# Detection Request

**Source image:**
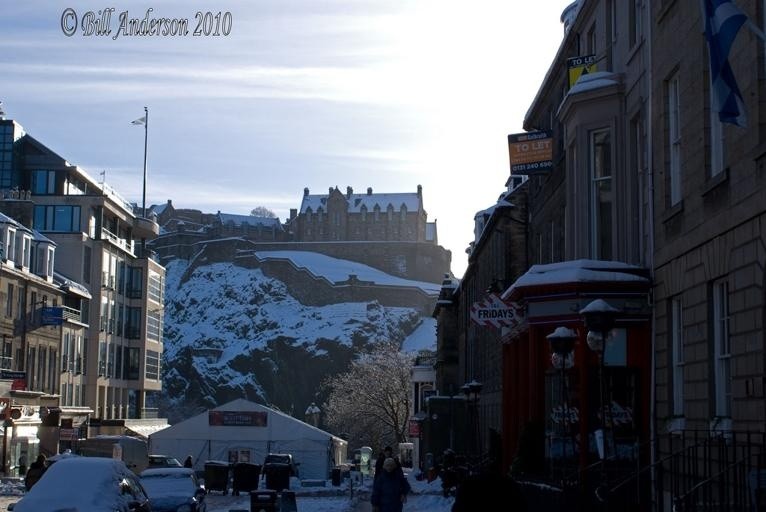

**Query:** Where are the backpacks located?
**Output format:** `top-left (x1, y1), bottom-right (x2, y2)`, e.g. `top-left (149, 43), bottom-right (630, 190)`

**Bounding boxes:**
top-left (25, 464), bottom-right (46, 489)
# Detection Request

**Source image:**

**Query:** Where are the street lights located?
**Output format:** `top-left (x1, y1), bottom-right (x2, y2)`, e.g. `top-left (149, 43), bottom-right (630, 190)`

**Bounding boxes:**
top-left (547, 327), bottom-right (580, 457)
top-left (461, 380), bottom-right (483, 463)
top-left (580, 299), bottom-right (620, 460)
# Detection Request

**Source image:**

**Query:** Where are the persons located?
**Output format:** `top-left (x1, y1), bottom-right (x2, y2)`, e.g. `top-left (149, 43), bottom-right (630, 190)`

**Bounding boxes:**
top-left (24, 454), bottom-right (48, 492)
top-left (183, 455), bottom-right (192, 468)
top-left (374, 446), bottom-right (393, 488)
top-left (369, 456), bottom-right (411, 512)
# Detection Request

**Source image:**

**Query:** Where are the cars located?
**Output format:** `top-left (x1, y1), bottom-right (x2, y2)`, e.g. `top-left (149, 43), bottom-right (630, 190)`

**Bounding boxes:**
top-left (8, 454), bottom-right (206, 512)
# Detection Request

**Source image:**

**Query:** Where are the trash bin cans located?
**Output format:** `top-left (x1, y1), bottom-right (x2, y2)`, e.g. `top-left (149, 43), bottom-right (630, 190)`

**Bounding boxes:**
top-left (232, 462), bottom-right (261, 496)
top-left (332, 469), bottom-right (340, 486)
top-left (251, 491), bottom-right (298, 512)
top-left (265, 463), bottom-right (289, 491)
top-left (204, 461), bottom-right (230, 496)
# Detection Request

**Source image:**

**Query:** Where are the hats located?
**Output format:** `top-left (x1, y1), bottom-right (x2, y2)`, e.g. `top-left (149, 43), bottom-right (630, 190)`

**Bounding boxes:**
top-left (384, 458), bottom-right (395, 467)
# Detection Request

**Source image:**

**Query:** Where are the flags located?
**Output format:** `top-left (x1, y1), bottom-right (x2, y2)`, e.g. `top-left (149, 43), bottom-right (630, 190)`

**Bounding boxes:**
top-left (693, 1), bottom-right (753, 129)
